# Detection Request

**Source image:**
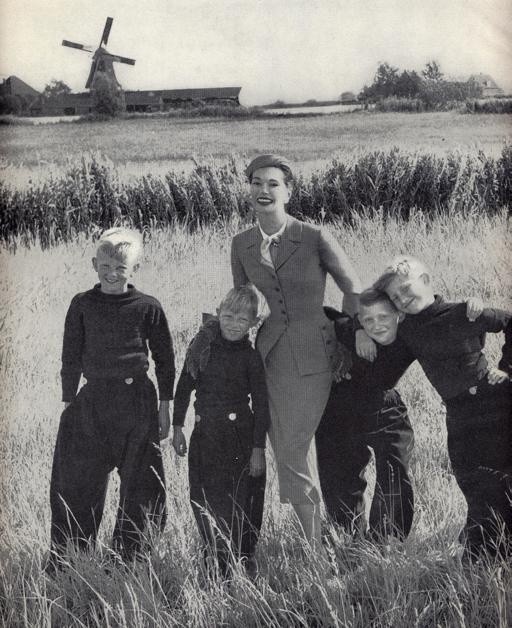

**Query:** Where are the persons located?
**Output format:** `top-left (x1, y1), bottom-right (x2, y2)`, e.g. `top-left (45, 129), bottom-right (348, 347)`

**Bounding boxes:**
top-left (314, 286), bottom-right (486, 560)
top-left (37, 223), bottom-right (176, 578)
top-left (167, 284), bottom-right (273, 579)
top-left (331, 256), bottom-right (512, 568)
top-left (182, 151), bottom-right (365, 578)
